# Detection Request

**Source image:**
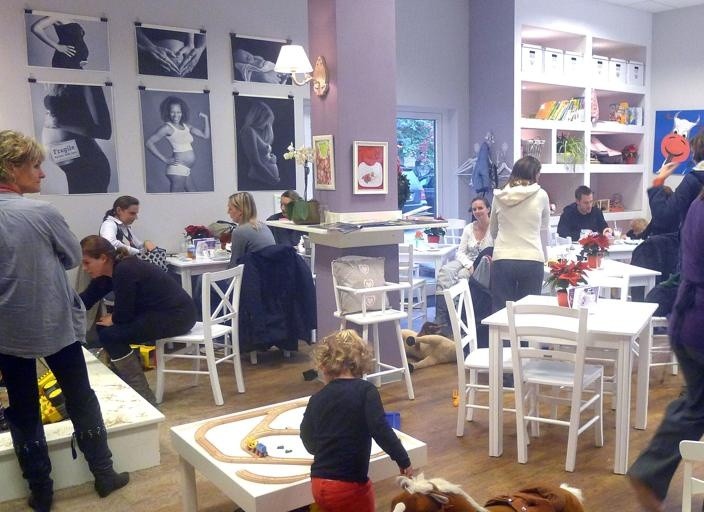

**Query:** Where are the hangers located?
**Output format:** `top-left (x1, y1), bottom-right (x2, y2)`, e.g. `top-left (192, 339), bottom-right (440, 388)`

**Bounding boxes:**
top-left (456, 153), bottom-right (513, 186)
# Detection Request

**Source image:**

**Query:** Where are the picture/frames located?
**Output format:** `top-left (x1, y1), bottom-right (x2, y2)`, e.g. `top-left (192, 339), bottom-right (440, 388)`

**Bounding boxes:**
top-left (353, 140), bottom-right (389, 195)
top-left (312, 135), bottom-right (335, 191)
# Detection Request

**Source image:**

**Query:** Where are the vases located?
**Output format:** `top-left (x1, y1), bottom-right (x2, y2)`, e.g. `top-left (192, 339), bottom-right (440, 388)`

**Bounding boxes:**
top-left (304, 161), bottom-right (315, 201)
top-left (624, 157), bottom-right (636, 164)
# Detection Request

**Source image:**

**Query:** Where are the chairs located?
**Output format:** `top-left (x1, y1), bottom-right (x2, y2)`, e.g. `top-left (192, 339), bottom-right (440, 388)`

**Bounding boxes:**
top-left (632, 315), bottom-right (679, 376)
top-left (680, 441), bottom-right (704, 511)
top-left (99, 297), bottom-right (115, 317)
top-left (444, 279), bottom-right (541, 446)
top-left (506, 300), bottom-right (605, 472)
top-left (442, 220), bottom-right (466, 245)
top-left (154, 264), bottom-right (246, 407)
top-left (330, 255), bottom-right (415, 399)
top-left (398, 245), bottom-right (427, 330)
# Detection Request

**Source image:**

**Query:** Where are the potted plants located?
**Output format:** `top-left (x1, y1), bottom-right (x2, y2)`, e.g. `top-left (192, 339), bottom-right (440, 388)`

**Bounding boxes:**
top-left (556, 131), bottom-right (584, 164)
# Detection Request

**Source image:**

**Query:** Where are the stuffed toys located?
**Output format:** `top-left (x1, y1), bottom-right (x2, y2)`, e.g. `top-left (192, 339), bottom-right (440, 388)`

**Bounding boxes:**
top-left (403, 327), bottom-right (464, 371)
top-left (393, 474), bottom-right (585, 511)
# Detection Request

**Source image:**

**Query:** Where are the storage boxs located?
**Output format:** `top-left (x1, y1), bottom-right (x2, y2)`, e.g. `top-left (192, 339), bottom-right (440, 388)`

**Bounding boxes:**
top-left (522, 138), bottom-right (545, 163)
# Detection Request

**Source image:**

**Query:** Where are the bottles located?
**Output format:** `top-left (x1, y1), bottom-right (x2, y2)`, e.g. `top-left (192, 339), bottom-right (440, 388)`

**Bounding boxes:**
top-left (545, 240), bottom-right (574, 262)
top-left (182, 241), bottom-right (223, 262)
top-left (584, 227), bottom-right (626, 245)
top-left (616, 106), bottom-right (623, 122)
top-left (582, 287), bottom-right (597, 315)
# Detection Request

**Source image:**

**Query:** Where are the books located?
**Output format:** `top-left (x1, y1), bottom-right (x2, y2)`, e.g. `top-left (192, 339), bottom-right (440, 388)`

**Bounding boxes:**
top-left (537, 97), bottom-right (585, 122)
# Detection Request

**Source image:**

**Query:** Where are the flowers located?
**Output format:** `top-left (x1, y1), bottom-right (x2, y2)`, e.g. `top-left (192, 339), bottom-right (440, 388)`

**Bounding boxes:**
top-left (184, 224), bottom-right (213, 238)
top-left (622, 143), bottom-right (639, 160)
top-left (283, 141), bottom-right (316, 201)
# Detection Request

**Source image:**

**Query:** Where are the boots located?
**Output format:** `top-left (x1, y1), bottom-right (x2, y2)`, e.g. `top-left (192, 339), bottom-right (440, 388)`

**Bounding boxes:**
top-left (26, 475), bottom-right (54, 512)
top-left (92, 466), bottom-right (131, 500)
top-left (110, 347), bottom-right (157, 405)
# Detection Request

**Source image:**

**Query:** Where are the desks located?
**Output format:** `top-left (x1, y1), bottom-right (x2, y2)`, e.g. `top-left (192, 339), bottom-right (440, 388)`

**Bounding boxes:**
top-left (544, 244), bottom-right (663, 301)
top-left (404, 242), bottom-right (459, 304)
top-left (165, 252), bottom-right (232, 355)
top-left (480, 293), bottom-right (659, 475)
top-left (265, 220), bottom-right (449, 373)
top-left (169, 394), bottom-right (428, 512)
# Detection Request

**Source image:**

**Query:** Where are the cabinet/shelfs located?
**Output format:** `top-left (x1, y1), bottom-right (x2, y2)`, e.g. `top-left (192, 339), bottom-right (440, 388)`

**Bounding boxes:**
top-left (470, 0), bottom-right (651, 241)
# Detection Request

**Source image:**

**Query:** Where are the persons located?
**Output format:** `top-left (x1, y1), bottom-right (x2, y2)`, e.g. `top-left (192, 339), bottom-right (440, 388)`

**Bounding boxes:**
top-left (624, 128), bottom-right (704, 512)
top-left (489, 156), bottom-right (551, 313)
top-left (135, 21), bottom-right (208, 76)
top-left (100, 196), bottom-right (155, 300)
top-left (75, 235), bottom-right (197, 407)
top-left (147, 93), bottom-right (210, 192)
top-left (430, 197), bottom-right (495, 329)
top-left (31, 12), bottom-right (88, 68)
top-left (235, 45), bottom-right (280, 84)
top-left (41, 84), bottom-right (113, 193)
top-left (558, 186), bottom-right (614, 243)
top-left (241, 99), bottom-right (281, 190)
top-left (194, 191), bottom-right (275, 306)
top-left (299, 329), bottom-right (413, 512)
top-left (0, 127), bottom-right (129, 512)
top-left (266, 190), bottom-right (304, 246)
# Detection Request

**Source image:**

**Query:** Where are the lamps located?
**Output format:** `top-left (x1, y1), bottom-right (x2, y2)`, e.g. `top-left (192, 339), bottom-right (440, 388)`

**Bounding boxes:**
top-left (275, 43), bottom-right (328, 98)
